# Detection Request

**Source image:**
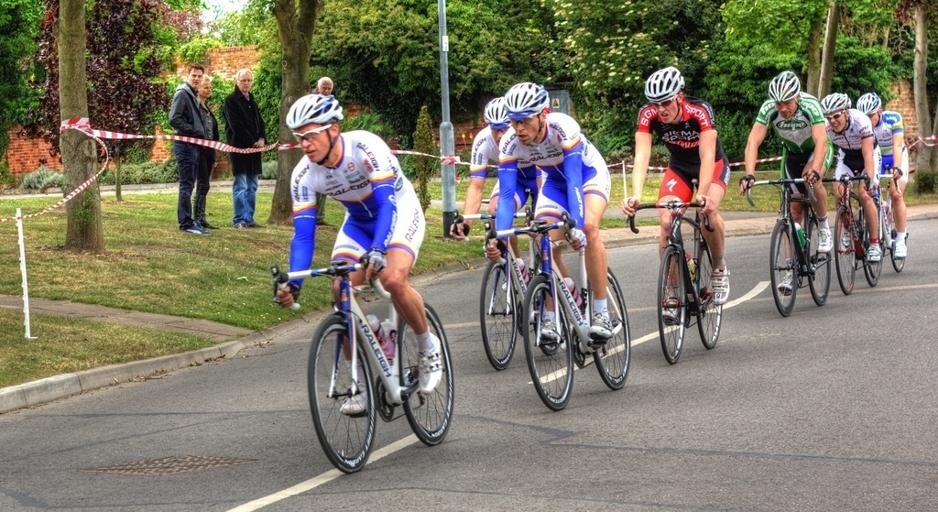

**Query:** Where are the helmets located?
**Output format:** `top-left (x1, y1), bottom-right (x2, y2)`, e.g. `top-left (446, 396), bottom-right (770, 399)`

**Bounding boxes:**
top-left (820, 93), bottom-right (852, 114)
top-left (768, 70), bottom-right (801, 103)
top-left (856, 91), bottom-right (882, 115)
top-left (483, 81), bottom-right (551, 129)
top-left (645, 66), bottom-right (685, 104)
top-left (285, 93), bottom-right (344, 130)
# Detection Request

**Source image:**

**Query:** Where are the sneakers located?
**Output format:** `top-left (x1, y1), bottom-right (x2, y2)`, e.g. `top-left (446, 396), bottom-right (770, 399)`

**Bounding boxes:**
top-left (895, 238), bottom-right (907, 257)
top-left (817, 229), bottom-right (833, 253)
top-left (234, 222), bottom-right (266, 230)
top-left (662, 296), bottom-right (680, 320)
top-left (541, 319), bottom-right (556, 339)
top-left (418, 333), bottom-right (442, 392)
top-left (777, 271), bottom-right (792, 296)
top-left (710, 266), bottom-right (730, 305)
top-left (842, 229), bottom-right (852, 250)
top-left (867, 245), bottom-right (881, 261)
top-left (186, 221), bottom-right (209, 234)
top-left (340, 383), bottom-right (367, 415)
top-left (589, 315), bottom-right (613, 339)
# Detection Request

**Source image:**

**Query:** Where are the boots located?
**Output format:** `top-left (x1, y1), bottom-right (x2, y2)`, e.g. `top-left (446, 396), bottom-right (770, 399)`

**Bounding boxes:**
top-left (193, 194), bottom-right (219, 233)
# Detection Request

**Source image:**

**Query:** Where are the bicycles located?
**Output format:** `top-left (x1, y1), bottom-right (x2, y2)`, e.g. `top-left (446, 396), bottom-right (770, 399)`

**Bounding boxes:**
top-left (449, 205), bottom-right (566, 371)
top-left (484, 211), bottom-right (633, 410)
top-left (742, 174), bottom-right (832, 318)
top-left (629, 198), bottom-right (724, 363)
top-left (820, 170), bottom-right (910, 296)
top-left (270, 254), bottom-right (453, 473)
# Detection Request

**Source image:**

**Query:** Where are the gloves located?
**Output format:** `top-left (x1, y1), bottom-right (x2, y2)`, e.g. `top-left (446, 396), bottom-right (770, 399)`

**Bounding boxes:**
top-left (803, 170), bottom-right (820, 185)
top-left (487, 237), bottom-right (508, 265)
top-left (565, 226), bottom-right (587, 251)
top-left (738, 174), bottom-right (755, 191)
top-left (890, 166), bottom-right (903, 179)
top-left (361, 248), bottom-right (385, 275)
top-left (449, 221), bottom-right (470, 242)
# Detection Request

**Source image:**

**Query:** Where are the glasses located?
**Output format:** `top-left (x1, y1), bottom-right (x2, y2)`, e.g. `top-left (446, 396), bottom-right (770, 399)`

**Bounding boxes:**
top-left (652, 95), bottom-right (678, 107)
top-left (292, 124), bottom-right (333, 140)
top-left (825, 110), bottom-right (847, 120)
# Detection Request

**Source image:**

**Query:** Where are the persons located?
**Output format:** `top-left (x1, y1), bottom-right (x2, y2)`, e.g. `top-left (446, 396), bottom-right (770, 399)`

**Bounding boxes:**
top-left (483, 81), bottom-right (614, 341)
top-left (737, 69), bottom-right (835, 292)
top-left (274, 92), bottom-right (446, 417)
top-left (620, 65), bottom-right (732, 320)
top-left (448, 96), bottom-right (581, 311)
top-left (166, 62), bottom-right (214, 238)
top-left (820, 92), bottom-right (884, 265)
top-left (286, 77), bottom-right (334, 226)
top-left (193, 73), bottom-right (222, 230)
top-left (220, 67), bottom-right (269, 230)
top-left (855, 91), bottom-right (911, 259)
